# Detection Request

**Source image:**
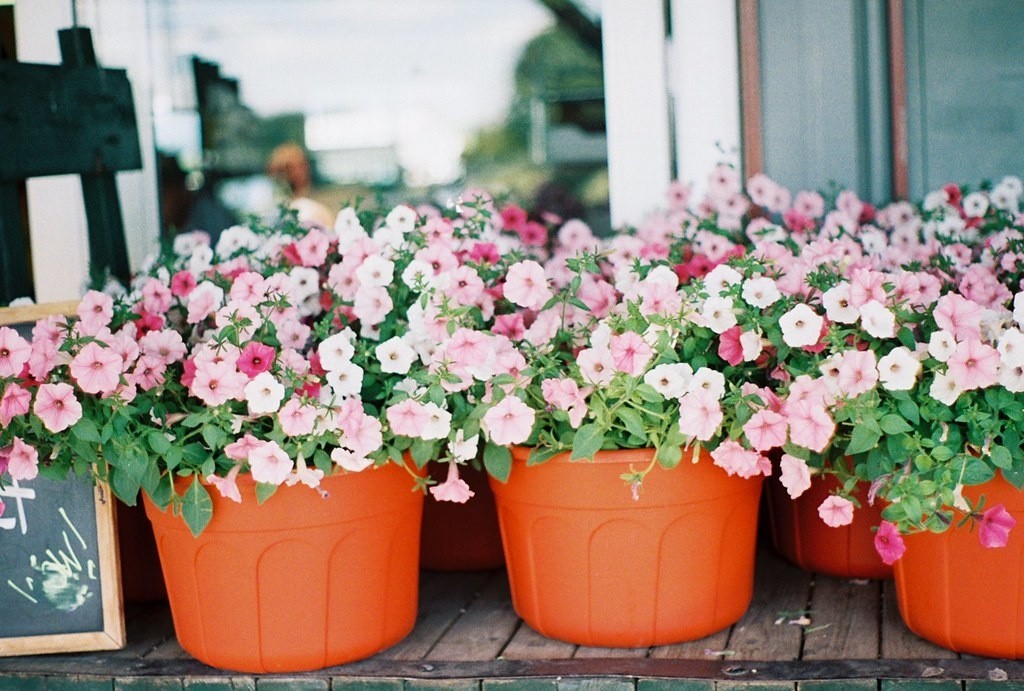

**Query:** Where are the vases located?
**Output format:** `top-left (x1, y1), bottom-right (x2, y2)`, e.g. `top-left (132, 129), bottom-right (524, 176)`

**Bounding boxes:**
top-left (114, 444), bottom-right (1024, 674)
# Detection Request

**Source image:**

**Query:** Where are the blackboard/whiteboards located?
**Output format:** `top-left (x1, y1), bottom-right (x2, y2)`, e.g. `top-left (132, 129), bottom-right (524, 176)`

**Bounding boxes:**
top-left (0, 300), bottom-right (125, 659)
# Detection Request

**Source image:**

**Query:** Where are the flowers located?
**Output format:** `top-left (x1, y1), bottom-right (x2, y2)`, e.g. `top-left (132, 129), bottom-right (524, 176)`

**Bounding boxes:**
top-left (0, 173), bottom-right (1024, 565)
